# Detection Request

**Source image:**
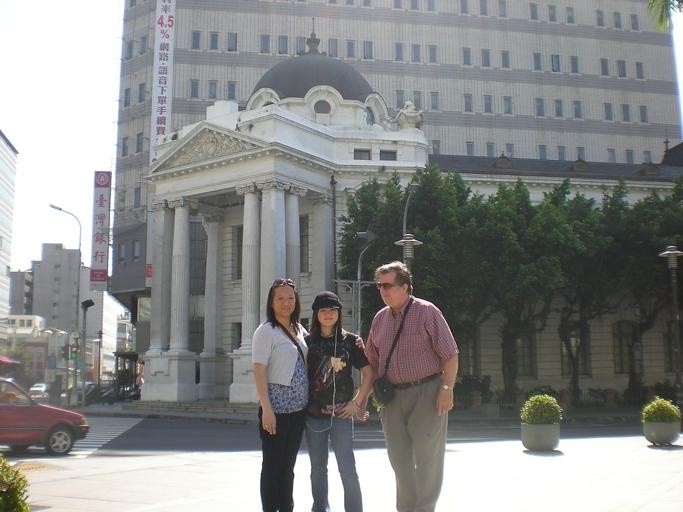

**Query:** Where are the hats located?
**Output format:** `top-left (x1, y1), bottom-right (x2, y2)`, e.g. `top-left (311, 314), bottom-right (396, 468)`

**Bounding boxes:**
top-left (311, 291), bottom-right (342, 309)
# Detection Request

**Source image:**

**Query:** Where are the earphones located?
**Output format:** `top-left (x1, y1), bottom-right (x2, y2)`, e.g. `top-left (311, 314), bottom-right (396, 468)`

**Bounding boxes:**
top-left (332, 410), bottom-right (335, 417)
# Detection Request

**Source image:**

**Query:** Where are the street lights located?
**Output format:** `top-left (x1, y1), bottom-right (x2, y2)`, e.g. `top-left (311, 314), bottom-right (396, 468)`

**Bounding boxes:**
top-left (400, 183), bottom-right (421, 237)
top-left (393, 232), bottom-right (422, 297)
top-left (49, 203), bottom-right (83, 406)
top-left (80, 298), bottom-right (94, 404)
top-left (658, 242), bottom-right (682, 412)
top-left (93, 327), bottom-right (104, 393)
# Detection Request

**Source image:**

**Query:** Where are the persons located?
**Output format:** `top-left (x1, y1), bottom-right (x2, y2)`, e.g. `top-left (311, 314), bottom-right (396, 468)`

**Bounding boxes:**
top-left (252, 278), bottom-right (365, 512)
top-left (304, 291), bottom-right (373, 512)
top-left (356, 260), bottom-right (460, 512)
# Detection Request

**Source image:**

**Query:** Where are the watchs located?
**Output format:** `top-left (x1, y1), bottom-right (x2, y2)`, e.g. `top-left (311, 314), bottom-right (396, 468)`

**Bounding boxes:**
top-left (440, 384), bottom-right (453, 391)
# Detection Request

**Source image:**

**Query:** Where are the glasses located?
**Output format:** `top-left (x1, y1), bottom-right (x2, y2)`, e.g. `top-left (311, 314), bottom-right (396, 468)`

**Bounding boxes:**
top-left (272, 278), bottom-right (296, 289)
top-left (375, 282), bottom-right (401, 289)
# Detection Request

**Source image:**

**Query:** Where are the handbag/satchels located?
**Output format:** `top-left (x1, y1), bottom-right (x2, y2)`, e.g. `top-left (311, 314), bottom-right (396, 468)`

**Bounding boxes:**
top-left (371, 379), bottom-right (394, 406)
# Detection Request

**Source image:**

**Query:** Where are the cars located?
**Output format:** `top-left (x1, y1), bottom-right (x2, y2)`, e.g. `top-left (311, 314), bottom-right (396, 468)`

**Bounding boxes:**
top-left (28, 377), bottom-right (118, 401)
top-left (0, 377), bottom-right (89, 456)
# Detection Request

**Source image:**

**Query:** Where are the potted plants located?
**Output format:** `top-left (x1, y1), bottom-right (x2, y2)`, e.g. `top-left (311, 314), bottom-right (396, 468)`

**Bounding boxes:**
top-left (520, 394), bottom-right (563, 451)
top-left (641, 397), bottom-right (682, 445)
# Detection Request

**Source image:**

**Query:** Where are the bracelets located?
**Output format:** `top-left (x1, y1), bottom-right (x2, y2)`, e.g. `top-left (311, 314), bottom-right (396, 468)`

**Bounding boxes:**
top-left (352, 399), bottom-right (360, 409)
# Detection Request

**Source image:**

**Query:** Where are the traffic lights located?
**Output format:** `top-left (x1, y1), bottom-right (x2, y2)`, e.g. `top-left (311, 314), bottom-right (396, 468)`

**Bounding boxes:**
top-left (70, 352), bottom-right (77, 359)
top-left (60, 344), bottom-right (67, 359)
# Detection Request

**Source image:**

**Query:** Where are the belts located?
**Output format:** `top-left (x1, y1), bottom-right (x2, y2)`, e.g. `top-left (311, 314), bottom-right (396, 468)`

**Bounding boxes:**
top-left (393, 370), bottom-right (443, 391)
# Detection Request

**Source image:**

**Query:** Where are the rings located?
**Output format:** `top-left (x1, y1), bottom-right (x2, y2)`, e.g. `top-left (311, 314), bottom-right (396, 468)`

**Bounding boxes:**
top-left (269, 424), bottom-right (272, 426)
